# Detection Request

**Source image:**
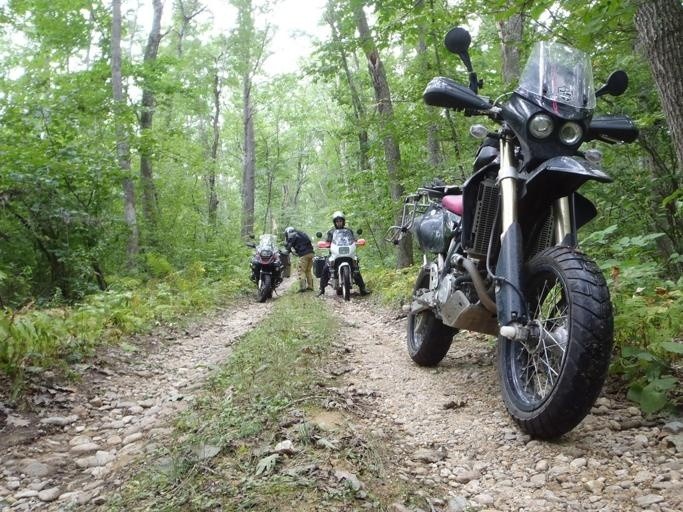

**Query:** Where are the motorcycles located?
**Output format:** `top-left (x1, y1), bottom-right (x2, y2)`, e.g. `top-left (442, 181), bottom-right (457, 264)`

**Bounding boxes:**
top-left (382, 21), bottom-right (650, 446)
top-left (310, 228), bottom-right (367, 303)
top-left (244, 233), bottom-right (290, 305)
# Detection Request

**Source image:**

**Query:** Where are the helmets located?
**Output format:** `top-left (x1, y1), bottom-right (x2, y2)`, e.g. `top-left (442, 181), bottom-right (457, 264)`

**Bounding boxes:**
top-left (333, 211), bottom-right (345, 229)
top-left (284, 227), bottom-right (295, 236)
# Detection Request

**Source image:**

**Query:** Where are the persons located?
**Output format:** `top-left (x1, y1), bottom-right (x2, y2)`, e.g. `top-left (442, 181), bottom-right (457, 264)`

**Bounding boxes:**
top-left (310, 210), bottom-right (372, 297)
top-left (282, 226), bottom-right (315, 294)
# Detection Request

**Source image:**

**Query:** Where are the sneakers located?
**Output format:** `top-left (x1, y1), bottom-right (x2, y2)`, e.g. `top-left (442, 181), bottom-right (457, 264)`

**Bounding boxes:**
top-left (360, 288), bottom-right (370, 295)
top-left (316, 290), bottom-right (324, 296)
